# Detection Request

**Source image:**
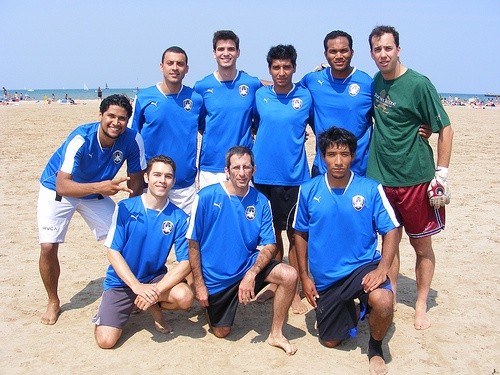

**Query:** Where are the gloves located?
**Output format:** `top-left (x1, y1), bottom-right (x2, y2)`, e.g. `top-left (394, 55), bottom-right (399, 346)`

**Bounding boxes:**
top-left (426, 165), bottom-right (451, 207)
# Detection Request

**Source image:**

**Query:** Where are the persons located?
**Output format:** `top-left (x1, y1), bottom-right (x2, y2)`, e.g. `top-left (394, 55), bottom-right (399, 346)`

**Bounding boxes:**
top-left (0, 84), bottom-right (76, 109)
top-left (37, 26), bottom-right (454, 374)
top-left (97, 86), bottom-right (102, 101)
top-left (433, 93), bottom-right (500, 112)
top-left (119, 91), bottom-right (134, 101)
